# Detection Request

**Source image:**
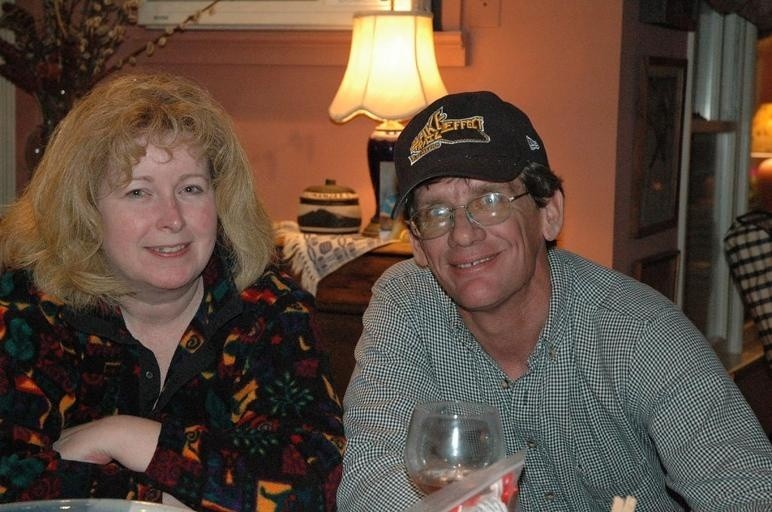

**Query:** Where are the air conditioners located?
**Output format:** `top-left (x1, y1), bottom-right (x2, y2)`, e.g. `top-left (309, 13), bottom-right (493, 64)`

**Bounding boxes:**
top-left (137, 0), bottom-right (429, 32)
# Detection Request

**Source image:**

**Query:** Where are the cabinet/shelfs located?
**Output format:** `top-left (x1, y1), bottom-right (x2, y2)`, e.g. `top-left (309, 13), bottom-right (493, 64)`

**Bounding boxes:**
top-left (271, 218), bottom-right (420, 402)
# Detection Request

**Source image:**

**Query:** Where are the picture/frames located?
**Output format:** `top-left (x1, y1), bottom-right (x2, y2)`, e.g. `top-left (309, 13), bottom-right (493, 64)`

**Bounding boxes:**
top-left (633, 249), bottom-right (680, 307)
top-left (630, 54), bottom-right (688, 241)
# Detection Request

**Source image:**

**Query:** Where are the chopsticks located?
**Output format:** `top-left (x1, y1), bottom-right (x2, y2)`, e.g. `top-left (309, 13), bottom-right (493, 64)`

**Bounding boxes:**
top-left (610, 495), bottom-right (638, 511)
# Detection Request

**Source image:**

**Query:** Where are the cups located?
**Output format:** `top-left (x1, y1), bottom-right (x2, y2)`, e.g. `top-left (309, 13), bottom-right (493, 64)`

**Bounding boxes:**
top-left (402, 400), bottom-right (505, 512)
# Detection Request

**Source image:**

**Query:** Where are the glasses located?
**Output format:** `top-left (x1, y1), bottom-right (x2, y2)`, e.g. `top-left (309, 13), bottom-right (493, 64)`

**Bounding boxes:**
top-left (409, 192), bottom-right (528, 240)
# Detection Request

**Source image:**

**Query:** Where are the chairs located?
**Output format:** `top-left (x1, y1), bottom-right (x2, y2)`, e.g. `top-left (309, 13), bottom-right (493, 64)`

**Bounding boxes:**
top-left (722, 211), bottom-right (772, 372)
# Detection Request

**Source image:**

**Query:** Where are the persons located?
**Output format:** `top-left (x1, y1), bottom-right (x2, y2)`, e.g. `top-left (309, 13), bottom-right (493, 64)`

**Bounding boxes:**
top-left (1, 69), bottom-right (347, 512)
top-left (337, 90), bottom-right (771, 512)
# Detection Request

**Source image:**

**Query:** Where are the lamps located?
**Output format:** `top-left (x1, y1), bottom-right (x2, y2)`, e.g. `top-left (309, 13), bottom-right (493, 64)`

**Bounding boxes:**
top-left (327, 10), bottom-right (449, 237)
top-left (750, 103), bottom-right (772, 210)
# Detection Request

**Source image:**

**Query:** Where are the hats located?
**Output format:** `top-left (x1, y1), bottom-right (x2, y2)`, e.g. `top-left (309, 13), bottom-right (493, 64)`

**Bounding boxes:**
top-left (391, 91), bottom-right (548, 220)
